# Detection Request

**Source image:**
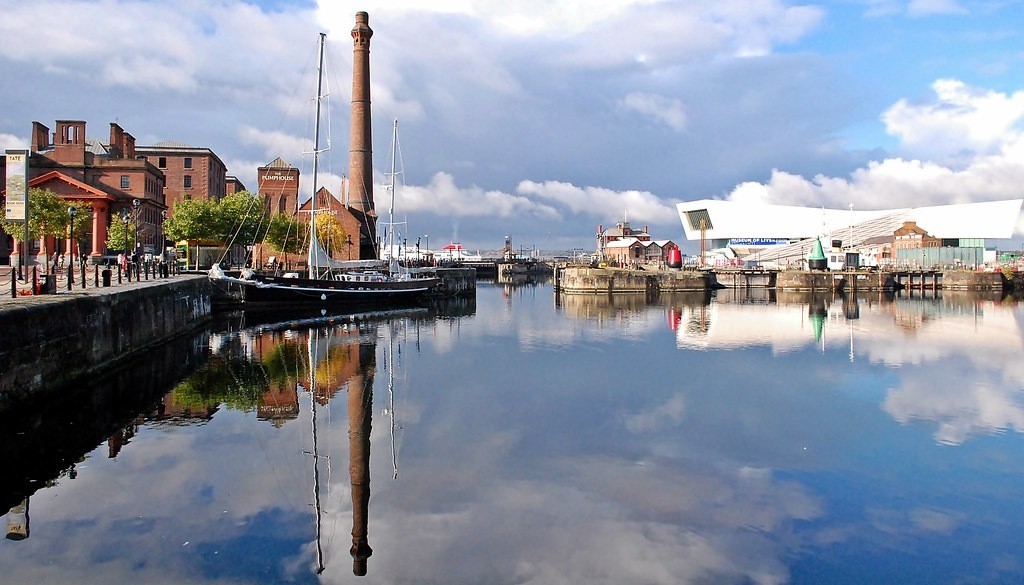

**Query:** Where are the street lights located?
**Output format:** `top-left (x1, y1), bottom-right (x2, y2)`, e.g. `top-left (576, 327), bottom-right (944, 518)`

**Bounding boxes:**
top-left (132, 199), bottom-right (141, 283)
top-left (66, 206), bottom-right (77, 290)
top-left (122, 206), bottom-right (131, 282)
top-left (161, 211), bottom-right (167, 276)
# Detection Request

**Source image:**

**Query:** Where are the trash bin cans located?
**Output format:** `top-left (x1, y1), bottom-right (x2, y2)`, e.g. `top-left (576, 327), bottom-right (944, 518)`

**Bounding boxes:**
top-left (41, 275), bottom-right (57, 294)
top-left (102, 269), bottom-right (113, 287)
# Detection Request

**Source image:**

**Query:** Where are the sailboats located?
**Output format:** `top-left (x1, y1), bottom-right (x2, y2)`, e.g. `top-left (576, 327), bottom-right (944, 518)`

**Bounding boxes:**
top-left (209, 30), bottom-right (441, 293)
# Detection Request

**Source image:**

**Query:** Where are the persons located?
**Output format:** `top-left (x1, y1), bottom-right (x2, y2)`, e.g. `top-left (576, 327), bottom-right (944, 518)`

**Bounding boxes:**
top-left (82, 252), bottom-right (88, 268)
top-left (396, 256), bottom-right (461, 267)
top-left (50, 251), bottom-right (59, 273)
top-left (117, 251), bottom-right (180, 274)
top-left (57, 252), bottom-right (64, 272)
